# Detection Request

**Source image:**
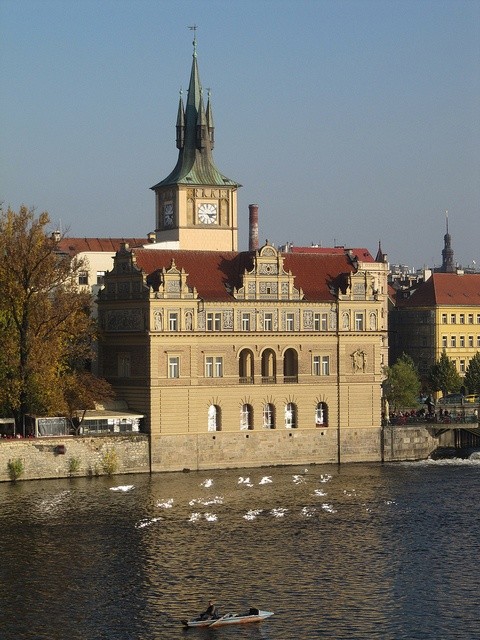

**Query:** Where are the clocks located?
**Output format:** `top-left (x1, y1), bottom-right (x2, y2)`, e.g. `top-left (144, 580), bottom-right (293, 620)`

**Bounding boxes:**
top-left (195, 200), bottom-right (218, 226)
top-left (163, 199), bottom-right (174, 225)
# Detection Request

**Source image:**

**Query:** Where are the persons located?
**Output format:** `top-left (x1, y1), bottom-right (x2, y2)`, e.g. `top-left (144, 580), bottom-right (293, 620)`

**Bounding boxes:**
top-left (199, 600), bottom-right (216, 620)
top-left (473, 409), bottom-right (478, 422)
top-left (388, 408), bottom-right (465, 424)
top-left (0, 431), bottom-right (34, 440)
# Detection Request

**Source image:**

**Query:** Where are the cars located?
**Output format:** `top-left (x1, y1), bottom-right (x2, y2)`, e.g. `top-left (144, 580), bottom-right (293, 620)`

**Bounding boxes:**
top-left (467, 394), bottom-right (479, 403)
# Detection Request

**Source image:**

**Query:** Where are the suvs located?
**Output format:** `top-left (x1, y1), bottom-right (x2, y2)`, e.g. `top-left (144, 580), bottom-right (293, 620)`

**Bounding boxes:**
top-left (439, 394), bottom-right (464, 403)
